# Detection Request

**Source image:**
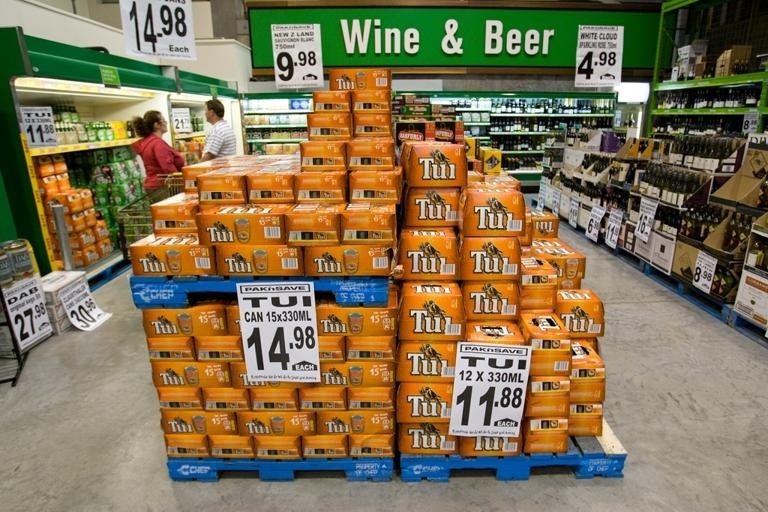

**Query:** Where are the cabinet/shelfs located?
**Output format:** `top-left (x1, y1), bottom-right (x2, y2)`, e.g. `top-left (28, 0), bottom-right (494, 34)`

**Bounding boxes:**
top-left (1, 27), bottom-right (247, 291)
top-left (239, 92), bottom-right (620, 187)
top-left (646, 0), bottom-right (768, 137)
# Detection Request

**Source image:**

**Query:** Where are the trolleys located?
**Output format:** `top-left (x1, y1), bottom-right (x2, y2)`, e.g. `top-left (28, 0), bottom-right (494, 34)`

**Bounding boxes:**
top-left (117, 173), bottom-right (184, 264)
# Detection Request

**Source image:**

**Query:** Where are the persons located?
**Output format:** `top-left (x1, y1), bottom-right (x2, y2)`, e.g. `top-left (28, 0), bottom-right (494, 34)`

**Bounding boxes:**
top-left (131, 111), bottom-right (184, 204)
top-left (200, 99), bottom-right (235, 162)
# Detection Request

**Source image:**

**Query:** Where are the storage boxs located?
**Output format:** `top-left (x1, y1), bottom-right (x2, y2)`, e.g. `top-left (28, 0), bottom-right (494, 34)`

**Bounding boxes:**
top-left (128, 69), bottom-right (607, 458)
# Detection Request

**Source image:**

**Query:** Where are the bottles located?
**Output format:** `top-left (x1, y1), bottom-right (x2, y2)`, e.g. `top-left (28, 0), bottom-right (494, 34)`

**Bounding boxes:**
top-left (489, 97), bottom-right (544, 169)
top-left (544, 59), bottom-right (767, 296)
top-left (126, 121), bottom-right (133, 137)
top-left (52, 105), bottom-right (113, 144)
